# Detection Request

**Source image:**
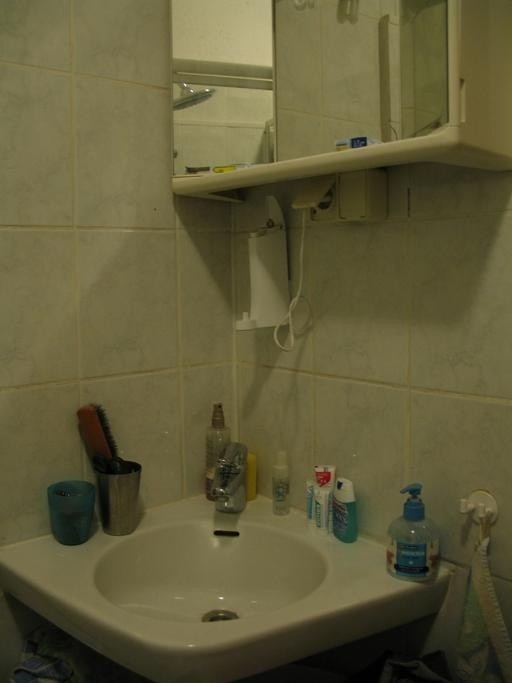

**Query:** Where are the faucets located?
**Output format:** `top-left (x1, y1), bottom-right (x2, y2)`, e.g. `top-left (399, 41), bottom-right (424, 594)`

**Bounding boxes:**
top-left (209, 440), bottom-right (247, 513)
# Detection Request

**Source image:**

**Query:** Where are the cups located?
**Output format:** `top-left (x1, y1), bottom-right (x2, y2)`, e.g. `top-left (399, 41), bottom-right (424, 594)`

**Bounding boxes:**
top-left (47, 480), bottom-right (95, 546)
top-left (96, 460), bottom-right (142, 536)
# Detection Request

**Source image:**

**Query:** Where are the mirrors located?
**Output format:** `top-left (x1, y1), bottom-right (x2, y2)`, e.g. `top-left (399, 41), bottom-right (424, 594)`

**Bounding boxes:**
top-left (169, 1), bottom-right (449, 177)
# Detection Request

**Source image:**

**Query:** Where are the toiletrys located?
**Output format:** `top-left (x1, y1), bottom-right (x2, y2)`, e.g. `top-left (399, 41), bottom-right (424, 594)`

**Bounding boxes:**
top-left (272, 450), bottom-right (290, 516)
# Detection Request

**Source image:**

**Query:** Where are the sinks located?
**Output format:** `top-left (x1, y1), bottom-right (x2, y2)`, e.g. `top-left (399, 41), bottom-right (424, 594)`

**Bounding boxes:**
top-left (94, 518), bottom-right (330, 622)
